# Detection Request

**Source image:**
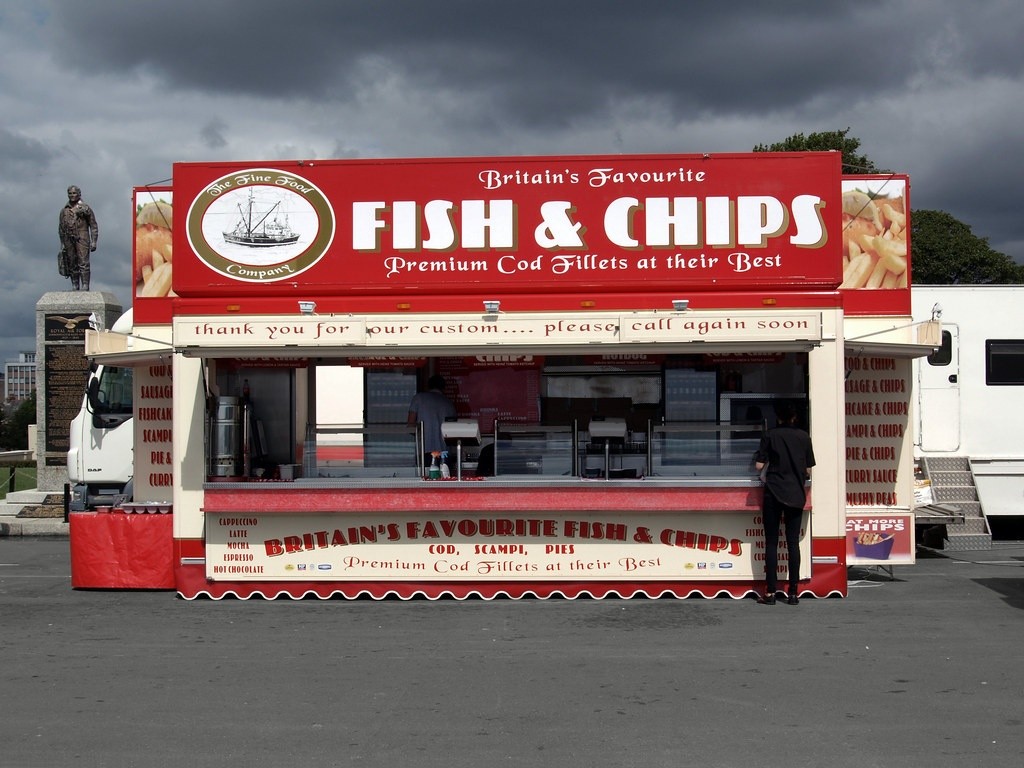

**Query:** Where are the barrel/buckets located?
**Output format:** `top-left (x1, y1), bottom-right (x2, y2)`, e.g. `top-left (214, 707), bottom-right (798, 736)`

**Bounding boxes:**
top-left (209, 395), bottom-right (243, 478)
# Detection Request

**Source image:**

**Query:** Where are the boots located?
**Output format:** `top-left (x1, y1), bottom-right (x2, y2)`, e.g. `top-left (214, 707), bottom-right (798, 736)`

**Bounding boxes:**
top-left (81, 271), bottom-right (91, 291)
top-left (72, 273), bottom-right (79, 290)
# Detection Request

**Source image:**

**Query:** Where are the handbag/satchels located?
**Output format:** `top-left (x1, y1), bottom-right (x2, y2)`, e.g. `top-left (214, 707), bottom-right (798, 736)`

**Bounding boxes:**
top-left (57, 248), bottom-right (69, 278)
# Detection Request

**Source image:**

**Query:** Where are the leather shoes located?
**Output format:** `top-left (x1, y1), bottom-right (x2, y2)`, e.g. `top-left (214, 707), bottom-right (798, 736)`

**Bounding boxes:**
top-left (758, 596), bottom-right (775, 604)
top-left (788, 595), bottom-right (800, 604)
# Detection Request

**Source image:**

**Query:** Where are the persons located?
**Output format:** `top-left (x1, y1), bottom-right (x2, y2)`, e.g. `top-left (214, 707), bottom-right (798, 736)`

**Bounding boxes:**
top-left (751, 398), bottom-right (817, 605)
top-left (57, 185), bottom-right (99, 292)
top-left (736, 403), bottom-right (768, 440)
top-left (406, 375), bottom-right (458, 478)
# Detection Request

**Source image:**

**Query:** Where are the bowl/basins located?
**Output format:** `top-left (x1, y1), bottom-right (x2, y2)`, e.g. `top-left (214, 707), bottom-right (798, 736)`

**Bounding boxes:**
top-left (852, 533), bottom-right (895, 560)
